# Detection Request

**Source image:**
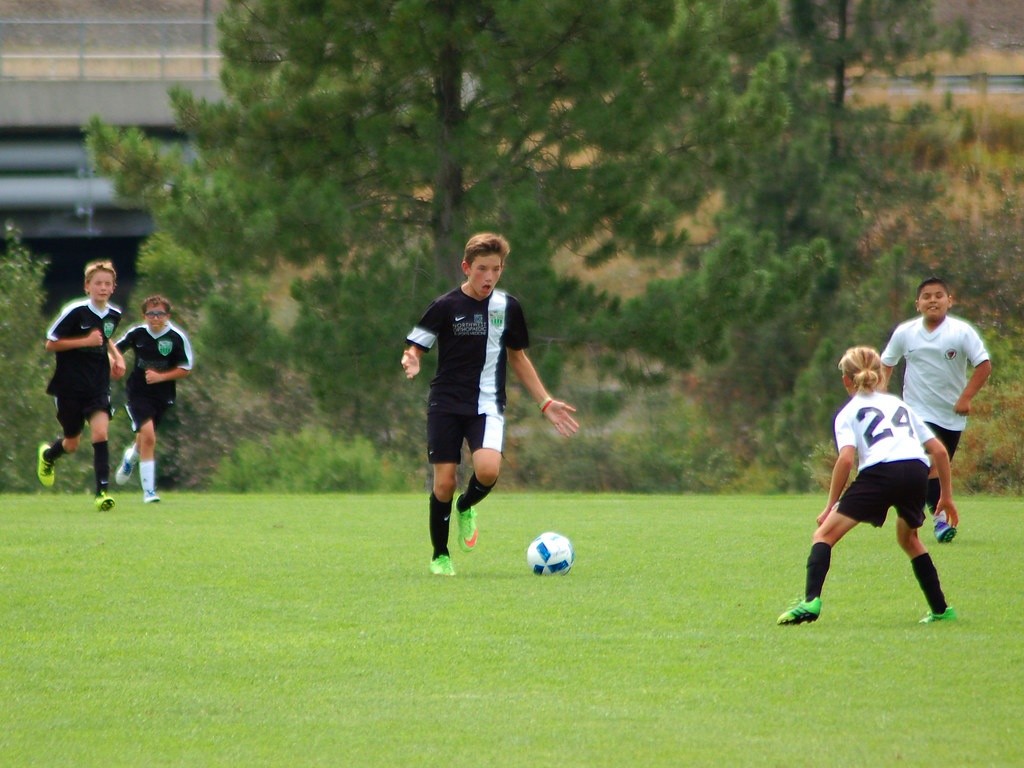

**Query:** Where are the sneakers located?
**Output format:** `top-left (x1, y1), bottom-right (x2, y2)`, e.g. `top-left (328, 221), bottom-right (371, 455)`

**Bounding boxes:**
top-left (144, 489), bottom-right (161, 503)
top-left (934, 521), bottom-right (957, 544)
top-left (429, 554), bottom-right (456, 576)
top-left (454, 494), bottom-right (477, 553)
top-left (37, 445), bottom-right (54, 487)
top-left (777, 597), bottom-right (822, 625)
top-left (918, 607), bottom-right (956, 623)
top-left (116, 448), bottom-right (137, 485)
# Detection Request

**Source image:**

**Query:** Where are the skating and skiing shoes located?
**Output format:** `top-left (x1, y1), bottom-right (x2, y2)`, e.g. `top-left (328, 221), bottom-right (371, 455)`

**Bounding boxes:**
top-left (96, 492), bottom-right (114, 510)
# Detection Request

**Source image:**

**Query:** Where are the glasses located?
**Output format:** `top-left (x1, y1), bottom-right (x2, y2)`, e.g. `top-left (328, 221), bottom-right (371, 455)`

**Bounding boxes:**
top-left (145, 311), bottom-right (167, 318)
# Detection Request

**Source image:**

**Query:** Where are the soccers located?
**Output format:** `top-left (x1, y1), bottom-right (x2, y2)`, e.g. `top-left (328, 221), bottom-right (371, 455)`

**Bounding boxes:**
top-left (525, 531), bottom-right (575, 577)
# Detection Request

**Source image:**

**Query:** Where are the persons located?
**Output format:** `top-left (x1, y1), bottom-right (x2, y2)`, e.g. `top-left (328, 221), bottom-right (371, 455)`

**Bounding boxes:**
top-left (108, 296), bottom-right (194, 503)
top-left (881, 278), bottom-right (991, 542)
top-left (401, 231), bottom-right (579, 575)
top-left (776, 346), bottom-right (958, 625)
top-left (38, 259), bottom-right (127, 511)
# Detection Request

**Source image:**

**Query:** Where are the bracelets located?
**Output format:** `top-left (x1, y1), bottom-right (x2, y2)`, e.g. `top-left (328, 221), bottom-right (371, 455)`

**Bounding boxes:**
top-left (537, 397), bottom-right (552, 413)
